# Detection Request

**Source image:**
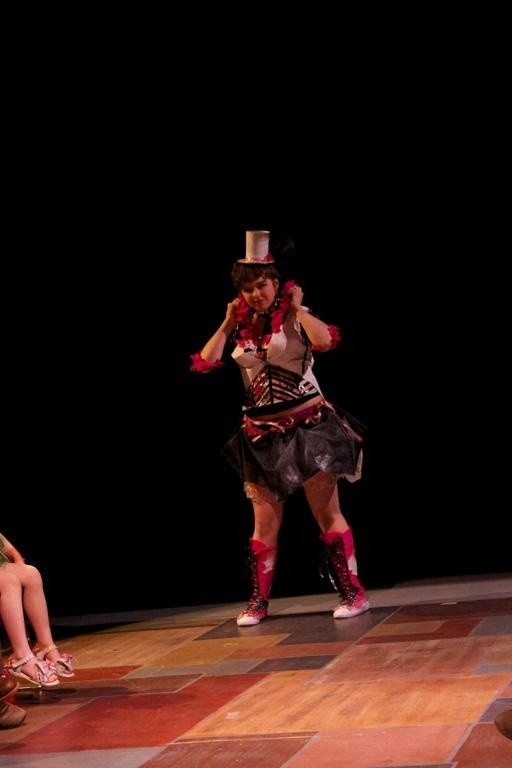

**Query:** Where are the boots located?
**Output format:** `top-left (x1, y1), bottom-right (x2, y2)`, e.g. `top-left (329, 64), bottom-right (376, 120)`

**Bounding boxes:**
top-left (318, 526), bottom-right (371, 620)
top-left (235, 538), bottom-right (278, 626)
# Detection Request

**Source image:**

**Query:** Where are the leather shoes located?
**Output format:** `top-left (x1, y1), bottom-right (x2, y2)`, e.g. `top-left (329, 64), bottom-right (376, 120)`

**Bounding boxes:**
top-left (0, 674), bottom-right (20, 703)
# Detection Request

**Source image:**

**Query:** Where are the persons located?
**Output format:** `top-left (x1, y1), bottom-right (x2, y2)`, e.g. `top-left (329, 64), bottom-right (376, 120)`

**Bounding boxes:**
top-left (1, 531), bottom-right (79, 690)
top-left (184, 261), bottom-right (370, 627)
top-left (0, 671), bottom-right (29, 730)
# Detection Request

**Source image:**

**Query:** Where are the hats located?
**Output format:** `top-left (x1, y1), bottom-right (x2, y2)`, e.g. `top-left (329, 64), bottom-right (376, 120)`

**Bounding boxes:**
top-left (237, 229), bottom-right (278, 264)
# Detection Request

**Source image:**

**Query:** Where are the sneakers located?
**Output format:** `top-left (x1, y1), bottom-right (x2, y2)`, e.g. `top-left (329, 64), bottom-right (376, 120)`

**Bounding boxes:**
top-left (0, 699), bottom-right (27, 730)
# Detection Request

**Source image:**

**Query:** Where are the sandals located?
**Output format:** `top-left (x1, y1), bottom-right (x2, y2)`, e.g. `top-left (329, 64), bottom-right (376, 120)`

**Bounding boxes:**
top-left (31, 644), bottom-right (75, 678)
top-left (5, 653), bottom-right (60, 687)
